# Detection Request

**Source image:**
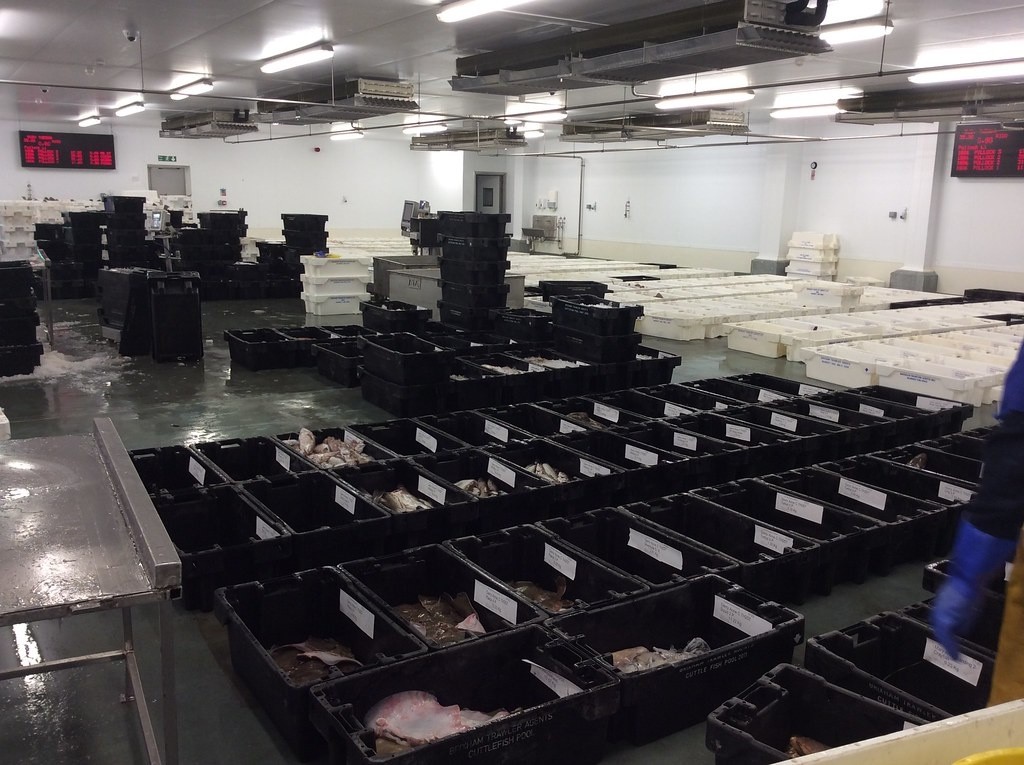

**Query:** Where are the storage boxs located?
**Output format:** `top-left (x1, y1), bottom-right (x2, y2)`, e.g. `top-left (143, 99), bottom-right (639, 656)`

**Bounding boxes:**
top-left (0, 190), bottom-right (1024, 765)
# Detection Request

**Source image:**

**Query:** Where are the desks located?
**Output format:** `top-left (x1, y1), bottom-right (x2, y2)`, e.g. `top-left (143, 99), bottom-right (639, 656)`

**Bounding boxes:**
top-left (0, 249), bottom-right (54, 352)
top-left (0, 418), bottom-right (183, 765)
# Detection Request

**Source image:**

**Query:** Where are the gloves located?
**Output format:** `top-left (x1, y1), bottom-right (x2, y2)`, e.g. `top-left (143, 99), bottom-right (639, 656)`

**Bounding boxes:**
top-left (934, 514), bottom-right (1018, 659)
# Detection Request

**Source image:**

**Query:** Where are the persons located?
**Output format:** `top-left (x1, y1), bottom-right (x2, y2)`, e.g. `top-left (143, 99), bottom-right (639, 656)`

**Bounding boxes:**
top-left (928, 338), bottom-right (1024, 664)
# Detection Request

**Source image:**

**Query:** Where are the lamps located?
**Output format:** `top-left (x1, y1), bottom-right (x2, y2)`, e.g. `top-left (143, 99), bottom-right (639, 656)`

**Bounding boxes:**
top-left (821, 18), bottom-right (894, 45)
top-left (259, 45), bottom-right (334, 74)
top-left (79, 118), bottom-right (100, 127)
top-left (170, 80), bottom-right (214, 101)
top-left (116, 102), bottom-right (145, 117)
top-left (655, 89), bottom-right (755, 109)
top-left (436, 0), bottom-right (535, 23)
top-left (771, 106), bottom-right (850, 119)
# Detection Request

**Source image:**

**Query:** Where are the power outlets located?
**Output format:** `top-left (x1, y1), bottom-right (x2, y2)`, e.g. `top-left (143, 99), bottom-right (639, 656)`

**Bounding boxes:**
top-left (343, 195), bottom-right (352, 203)
